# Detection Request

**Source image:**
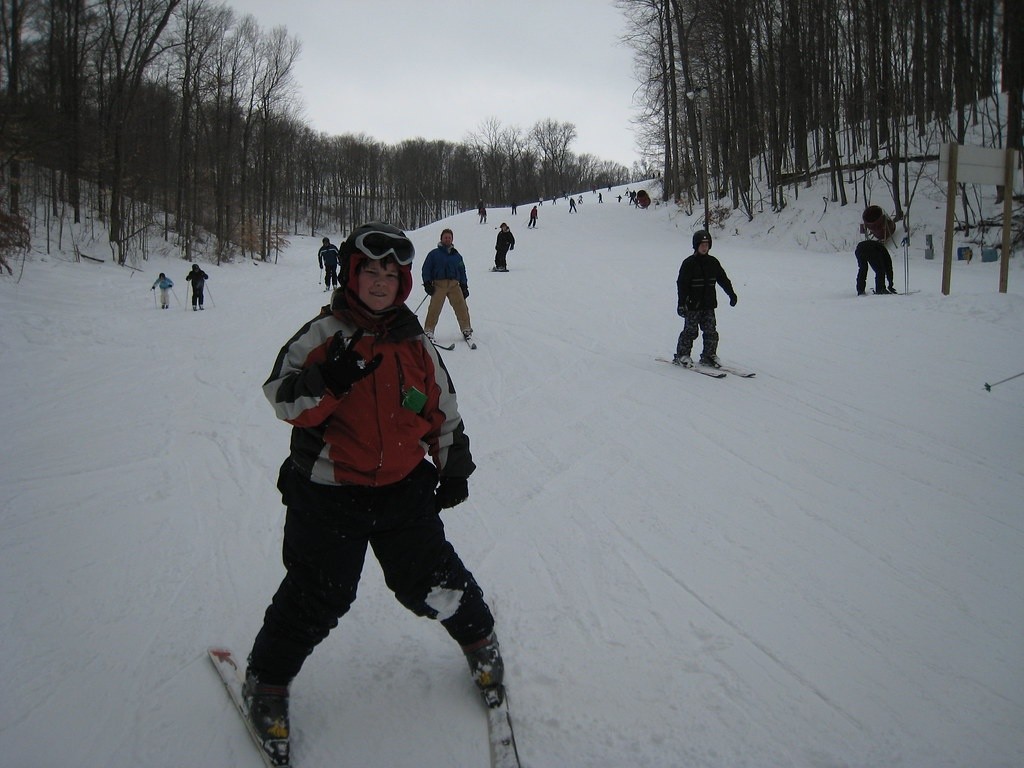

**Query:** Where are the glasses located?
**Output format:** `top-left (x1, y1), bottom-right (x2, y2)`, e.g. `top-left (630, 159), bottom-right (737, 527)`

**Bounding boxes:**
top-left (356, 231), bottom-right (414, 265)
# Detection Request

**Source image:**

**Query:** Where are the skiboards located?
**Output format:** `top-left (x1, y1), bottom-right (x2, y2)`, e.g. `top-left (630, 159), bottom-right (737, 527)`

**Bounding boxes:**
top-left (872, 289), bottom-right (921, 296)
top-left (431, 335), bottom-right (478, 350)
top-left (655, 357), bottom-right (756, 378)
top-left (528, 227), bottom-right (538, 229)
top-left (323, 289), bottom-right (336, 293)
top-left (209, 645), bottom-right (520, 768)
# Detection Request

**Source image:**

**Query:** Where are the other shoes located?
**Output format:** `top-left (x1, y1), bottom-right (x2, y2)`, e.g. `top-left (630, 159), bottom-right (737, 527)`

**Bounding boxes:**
top-left (674, 353), bottom-right (693, 368)
top-left (199, 304), bottom-right (204, 310)
top-left (701, 352), bottom-right (720, 367)
top-left (193, 306), bottom-right (197, 311)
top-left (463, 331), bottom-right (472, 339)
top-left (426, 335), bottom-right (436, 343)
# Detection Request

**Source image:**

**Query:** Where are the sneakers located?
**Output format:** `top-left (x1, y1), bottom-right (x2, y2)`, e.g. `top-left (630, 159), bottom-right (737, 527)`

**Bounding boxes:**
top-left (463, 629), bottom-right (503, 689)
top-left (242, 667), bottom-right (289, 740)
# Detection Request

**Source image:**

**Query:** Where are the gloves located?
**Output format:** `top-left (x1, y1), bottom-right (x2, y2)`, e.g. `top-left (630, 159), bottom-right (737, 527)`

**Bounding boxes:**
top-left (435, 476), bottom-right (468, 514)
top-left (320, 264), bottom-right (323, 269)
top-left (323, 328), bottom-right (383, 387)
top-left (495, 245), bottom-right (498, 250)
top-left (424, 281), bottom-right (432, 295)
top-left (510, 245), bottom-right (513, 250)
top-left (729, 293), bottom-right (738, 307)
top-left (461, 285), bottom-right (469, 299)
top-left (678, 303), bottom-right (688, 317)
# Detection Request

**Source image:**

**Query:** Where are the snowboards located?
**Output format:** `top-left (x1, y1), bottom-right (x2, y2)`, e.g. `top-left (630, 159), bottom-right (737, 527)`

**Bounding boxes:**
top-left (492, 268), bottom-right (509, 272)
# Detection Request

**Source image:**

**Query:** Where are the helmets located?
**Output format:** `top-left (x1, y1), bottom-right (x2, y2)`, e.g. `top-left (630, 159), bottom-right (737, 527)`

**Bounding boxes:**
top-left (339, 223), bottom-right (412, 288)
top-left (693, 230), bottom-right (712, 251)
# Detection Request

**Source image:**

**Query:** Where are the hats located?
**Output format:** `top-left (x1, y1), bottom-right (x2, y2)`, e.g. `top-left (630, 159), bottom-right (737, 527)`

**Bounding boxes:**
top-left (323, 238), bottom-right (329, 243)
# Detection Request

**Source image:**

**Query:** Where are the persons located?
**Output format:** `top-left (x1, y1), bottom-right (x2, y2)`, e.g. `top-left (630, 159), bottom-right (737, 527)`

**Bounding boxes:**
top-left (568, 197), bottom-right (577, 213)
top-left (597, 193), bottom-right (603, 203)
top-left (510, 199), bottom-right (517, 215)
top-left (591, 183), bottom-right (596, 194)
top-left (629, 190), bottom-right (639, 208)
top-left (242, 222), bottom-right (506, 758)
top-left (528, 206), bottom-right (538, 227)
top-left (673, 230), bottom-right (737, 368)
top-left (186, 264), bottom-right (208, 311)
top-left (494, 223), bottom-right (515, 271)
top-left (422, 229), bottom-right (474, 342)
top-left (318, 237), bottom-right (341, 292)
top-left (477, 199), bottom-right (487, 224)
top-left (624, 186), bottom-right (629, 196)
top-left (854, 240), bottom-right (898, 295)
top-left (615, 195), bottom-right (622, 204)
top-left (552, 194), bottom-right (556, 206)
top-left (538, 195), bottom-right (543, 206)
top-left (577, 194), bottom-right (583, 204)
top-left (606, 182), bottom-right (612, 191)
top-left (152, 273), bottom-right (174, 309)
top-left (564, 192), bottom-right (569, 200)
